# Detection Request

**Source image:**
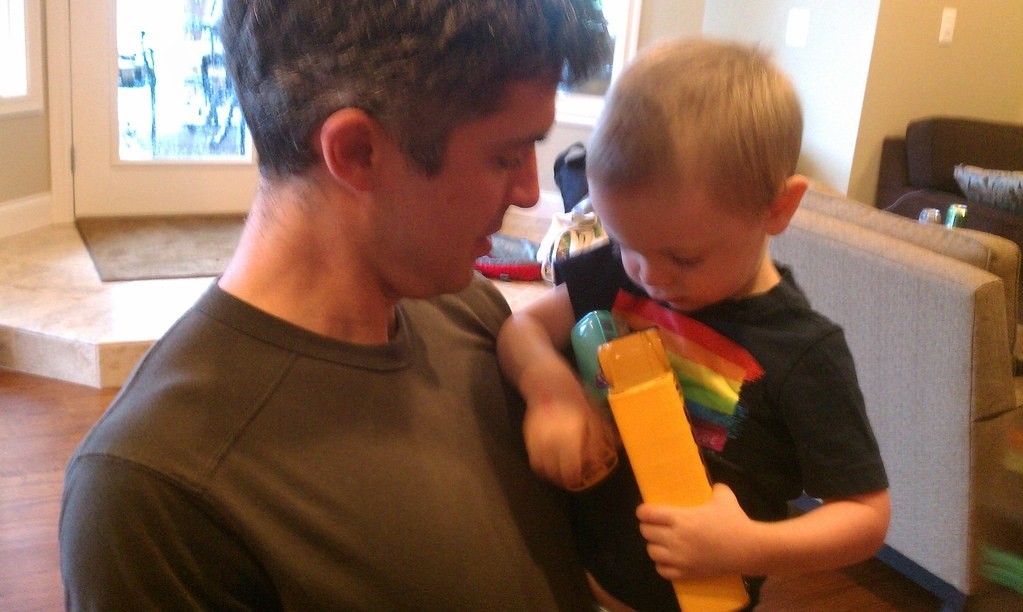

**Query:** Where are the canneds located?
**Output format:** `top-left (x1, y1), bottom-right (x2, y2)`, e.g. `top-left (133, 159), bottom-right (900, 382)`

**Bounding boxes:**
top-left (944, 204), bottom-right (968, 228)
top-left (918, 208), bottom-right (942, 224)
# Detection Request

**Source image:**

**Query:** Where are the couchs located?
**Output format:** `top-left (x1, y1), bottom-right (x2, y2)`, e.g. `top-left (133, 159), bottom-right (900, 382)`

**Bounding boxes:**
top-left (768, 115), bottom-right (1023, 612)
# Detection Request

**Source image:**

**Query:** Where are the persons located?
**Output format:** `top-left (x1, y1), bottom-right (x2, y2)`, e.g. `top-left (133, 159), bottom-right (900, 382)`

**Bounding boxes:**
top-left (495, 37), bottom-right (892, 612)
top-left (59, 0), bottom-right (604, 611)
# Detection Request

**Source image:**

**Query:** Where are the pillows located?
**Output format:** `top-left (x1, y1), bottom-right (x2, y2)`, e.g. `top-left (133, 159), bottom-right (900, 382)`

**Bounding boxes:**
top-left (954, 163), bottom-right (1023, 214)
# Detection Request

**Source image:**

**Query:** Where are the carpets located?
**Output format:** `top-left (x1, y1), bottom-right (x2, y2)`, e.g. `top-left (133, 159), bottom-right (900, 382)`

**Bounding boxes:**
top-left (76, 213), bottom-right (248, 281)
